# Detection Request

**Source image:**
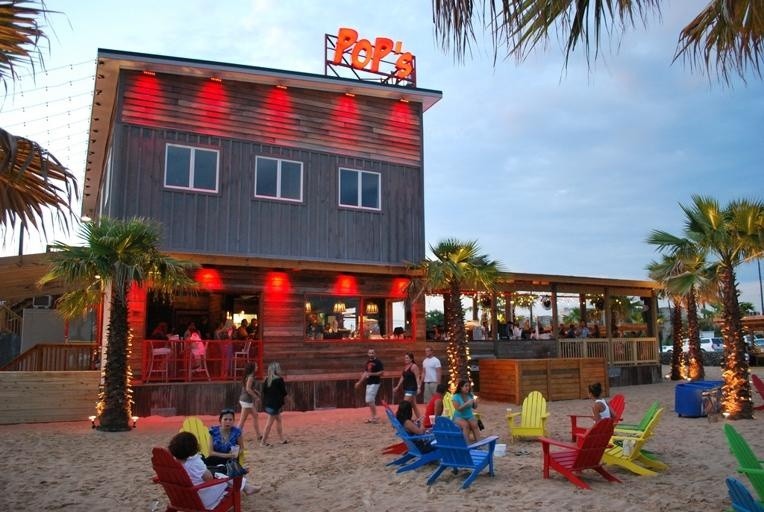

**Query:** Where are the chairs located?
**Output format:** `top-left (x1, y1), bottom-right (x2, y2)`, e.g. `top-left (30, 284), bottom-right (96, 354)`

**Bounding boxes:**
top-left (151, 446), bottom-right (243, 512)
top-left (179, 416), bottom-right (247, 498)
top-left (725, 476), bottom-right (764, 512)
top-left (674, 380), bottom-right (718, 418)
top-left (378, 389), bottom-right (669, 491)
top-left (692, 379), bottom-right (725, 389)
top-left (723, 423), bottom-right (764, 502)
top-left (145, 334), bottom-right (252, 385)
top-left (751, 373), bottom-right (764, 411)
top-left (433, 322), bottom-right (646, 342)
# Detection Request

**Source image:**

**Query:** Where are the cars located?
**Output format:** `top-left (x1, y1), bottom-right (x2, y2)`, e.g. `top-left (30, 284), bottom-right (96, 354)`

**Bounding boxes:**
top-left (660, 333), bottom-right (764, 354)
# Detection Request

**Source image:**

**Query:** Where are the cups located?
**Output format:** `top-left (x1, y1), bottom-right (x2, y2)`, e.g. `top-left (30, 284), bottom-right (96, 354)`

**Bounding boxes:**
top-left (429, 414), bottom-right (435, 424)
top-left (230, 446), bottom-right (239, 458)
top-left (506, 408), bottom-right (512, 416)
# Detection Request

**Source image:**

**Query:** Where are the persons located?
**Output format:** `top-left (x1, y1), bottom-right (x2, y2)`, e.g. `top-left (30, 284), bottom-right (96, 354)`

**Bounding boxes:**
top-left (260, 362), bottom-right (290, 445)
top-left (421, 346), bottom-right (442, 407)
top-left (150, 319), bottom-right (214, 365)
top-left (612, 319), bottom-right (636, 338)
top-left (483, 319), bottom-right (599, 339)
top-left (203, 408), bottom-right (244, 478)
top-left (238, 319), bottom-right (257, 337)
top-left (238, 362), bottom-right (262, 441)
top-left (451, 379), bottom-right (480, 442)
top-left (588, 383), bottom-right (616, 425)
top-left (167, 430), bottom-right (262, 510)
top-left (306, 319), bottom-right (339, 339)
top-left (423, 384), bottom-right (445, 427)
top-left (353, 346), bottom-right (383, 424)
top-left (392, 352), bottom-right (423, 420)
top-left (395, 400), bottom-right (438, 446)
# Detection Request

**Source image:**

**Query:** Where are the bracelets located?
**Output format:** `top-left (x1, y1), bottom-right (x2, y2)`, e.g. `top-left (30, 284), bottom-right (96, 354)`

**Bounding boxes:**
top-left (416, 383), bottom-right (421, 388)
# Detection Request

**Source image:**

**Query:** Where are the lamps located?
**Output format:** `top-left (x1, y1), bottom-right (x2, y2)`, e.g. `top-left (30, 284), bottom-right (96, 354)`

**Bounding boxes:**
top-left (332, 297), bottom-right (347, 314)
top-left (302, 294), bottom-right (313, 312)
top-left (366, 298), bottom-right (380, 315)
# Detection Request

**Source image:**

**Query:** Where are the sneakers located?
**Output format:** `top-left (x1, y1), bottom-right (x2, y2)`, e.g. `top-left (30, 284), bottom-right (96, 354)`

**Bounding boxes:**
top-left (260, 441), bottom-right (274, 449)
top-left (245, 485), bottom-right (262, 497)
